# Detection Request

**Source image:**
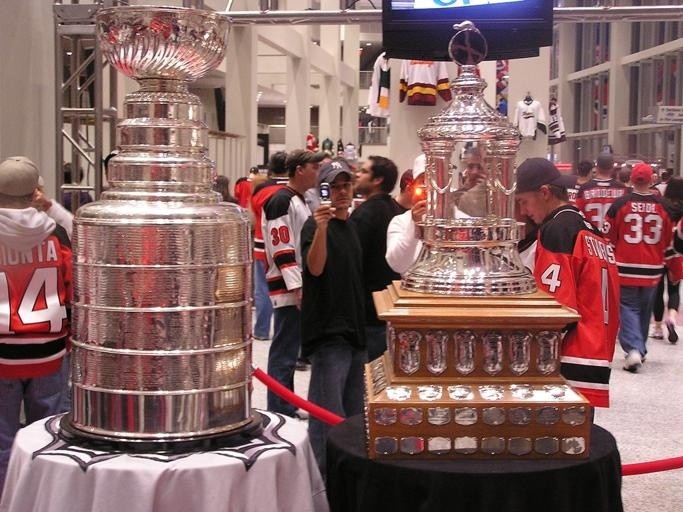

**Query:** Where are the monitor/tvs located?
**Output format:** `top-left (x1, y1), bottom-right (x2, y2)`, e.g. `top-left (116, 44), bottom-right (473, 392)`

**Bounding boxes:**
top-left (381, 0), bottom-right (555, 63)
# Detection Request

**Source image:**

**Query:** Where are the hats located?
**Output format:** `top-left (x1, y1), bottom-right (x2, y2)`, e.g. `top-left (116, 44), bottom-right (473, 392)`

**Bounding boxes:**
top-left (410, 153), bottom-right (426, 183)
top-left (268, 147), bottom-right (358, 193)
top-left (597, 150), bottom-right (676, 190)
top-left (0, 153), bottom-right (45, 200)
top-left (513, 156), bottom-right (580, 194)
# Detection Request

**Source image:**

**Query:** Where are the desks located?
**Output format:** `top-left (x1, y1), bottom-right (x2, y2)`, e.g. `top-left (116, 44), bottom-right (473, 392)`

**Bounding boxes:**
top-left (0, 408), bottom-right (310, 511)
top-left (324, 411), bottom-right (618, 512)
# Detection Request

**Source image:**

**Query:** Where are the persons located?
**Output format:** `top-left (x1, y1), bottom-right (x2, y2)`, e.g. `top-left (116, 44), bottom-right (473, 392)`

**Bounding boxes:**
top-left (382, 148), bottom-right (683, 426)
top-left (62, 163), bottom-right (92, 213)
top-left (212, 149), bottom-right (430, 481)
top-left (1, 155), bottom-right (78, 492)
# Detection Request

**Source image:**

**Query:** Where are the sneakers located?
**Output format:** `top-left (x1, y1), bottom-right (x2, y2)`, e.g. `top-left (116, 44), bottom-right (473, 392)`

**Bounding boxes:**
top-left (648, 318), bottom-right (680, 345)
top-left (618, 346), bottom-right (653, 377)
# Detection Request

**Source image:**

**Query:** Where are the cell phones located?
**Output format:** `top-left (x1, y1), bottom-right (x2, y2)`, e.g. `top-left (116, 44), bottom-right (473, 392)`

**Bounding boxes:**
top-left (319, 180), bottom-right (333, 211)
